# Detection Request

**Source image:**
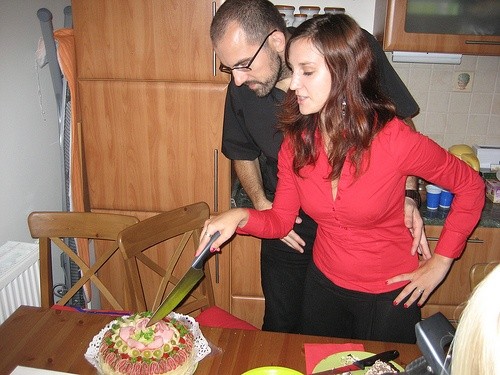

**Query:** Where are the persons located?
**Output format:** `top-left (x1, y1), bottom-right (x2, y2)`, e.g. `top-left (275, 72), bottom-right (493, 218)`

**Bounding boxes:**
top-left (450, 264), bottom-right (500, 375)
top-left (194, 13), bottom-right (485, 345)
top-left (209, 0), bottom-right (432, 335)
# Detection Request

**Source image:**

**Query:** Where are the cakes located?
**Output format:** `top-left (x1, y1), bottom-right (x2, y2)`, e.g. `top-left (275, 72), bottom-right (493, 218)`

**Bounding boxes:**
top-left (98, 312), bottom-right (195, 375)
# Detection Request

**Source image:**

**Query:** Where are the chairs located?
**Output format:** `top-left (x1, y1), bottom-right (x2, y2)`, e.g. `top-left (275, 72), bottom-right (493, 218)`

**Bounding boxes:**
top-left (27, 201), bottom-right (267, 331)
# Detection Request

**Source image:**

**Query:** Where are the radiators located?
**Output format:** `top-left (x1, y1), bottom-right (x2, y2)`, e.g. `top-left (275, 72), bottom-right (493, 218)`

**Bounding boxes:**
top-left (0, 238), bottom-right (42, 326)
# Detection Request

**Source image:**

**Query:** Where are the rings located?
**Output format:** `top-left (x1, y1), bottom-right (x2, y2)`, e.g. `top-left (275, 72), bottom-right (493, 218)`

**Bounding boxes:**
top-left (415, 288), bottom-right (423, 294)
top-left (204, 232), bottom-right (213, 238)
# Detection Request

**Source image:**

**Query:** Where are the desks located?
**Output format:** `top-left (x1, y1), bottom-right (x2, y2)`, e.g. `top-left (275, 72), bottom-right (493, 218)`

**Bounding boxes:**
top-left (0, 305), bottom-right (454, 375)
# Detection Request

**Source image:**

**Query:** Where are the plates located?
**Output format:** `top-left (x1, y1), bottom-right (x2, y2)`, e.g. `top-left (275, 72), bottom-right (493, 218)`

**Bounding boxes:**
top-left (311, 350), bottom-right (405, 375)
top-left (241, 366), bottom-right (304, 375)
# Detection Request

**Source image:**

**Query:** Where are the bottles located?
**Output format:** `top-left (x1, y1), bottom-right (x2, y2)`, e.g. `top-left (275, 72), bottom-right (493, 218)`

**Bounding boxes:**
top-left (275, 4), bottom-right (345, 29)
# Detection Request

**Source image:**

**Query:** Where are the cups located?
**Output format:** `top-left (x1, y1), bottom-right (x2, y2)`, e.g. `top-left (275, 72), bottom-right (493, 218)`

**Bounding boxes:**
top-left (426, 184), bottom-right (453, 210)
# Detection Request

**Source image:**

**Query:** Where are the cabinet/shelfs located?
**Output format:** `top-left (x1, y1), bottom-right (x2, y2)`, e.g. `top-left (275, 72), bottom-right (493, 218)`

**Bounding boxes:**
top-left (71, 0), bottom-right (500, 331)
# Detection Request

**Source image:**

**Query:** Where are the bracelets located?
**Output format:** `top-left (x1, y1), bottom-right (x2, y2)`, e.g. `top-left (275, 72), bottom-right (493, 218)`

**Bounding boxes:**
top-left (405, 190), bottom-right (422, 210)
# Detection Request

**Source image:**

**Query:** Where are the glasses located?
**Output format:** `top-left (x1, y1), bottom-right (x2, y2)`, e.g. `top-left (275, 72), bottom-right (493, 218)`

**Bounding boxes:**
top-left (218, 30), bottom-right (278, 74)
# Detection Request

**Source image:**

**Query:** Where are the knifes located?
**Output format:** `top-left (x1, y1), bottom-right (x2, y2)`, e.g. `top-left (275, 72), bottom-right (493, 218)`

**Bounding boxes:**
top-left (312, 350), bottom-right (400, 375)
top-left (144, 232), bottom-right (220, 327)
